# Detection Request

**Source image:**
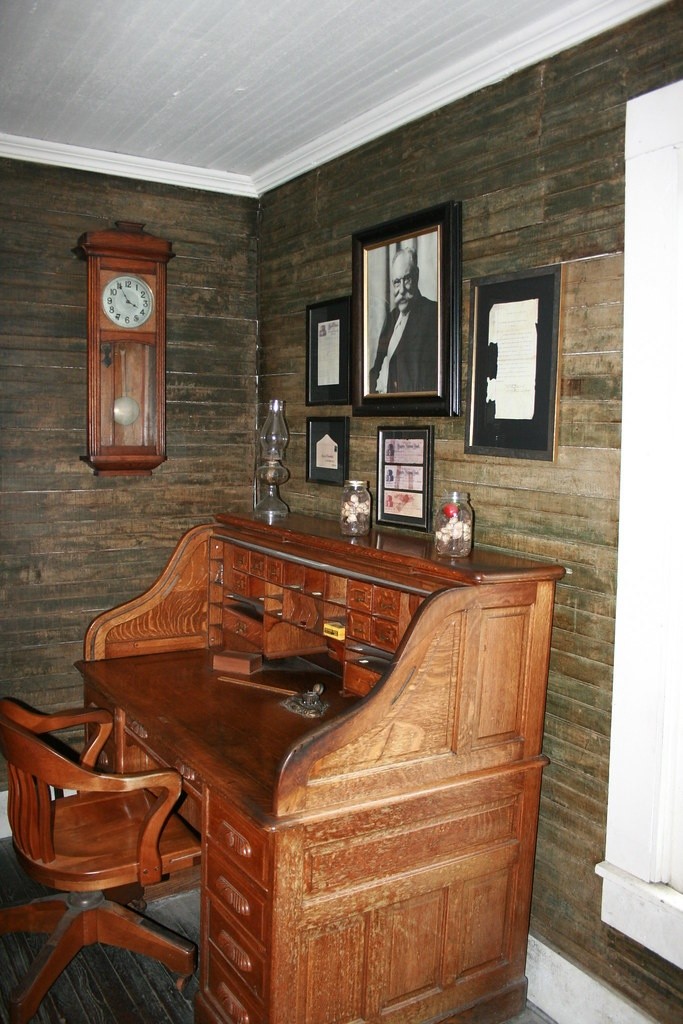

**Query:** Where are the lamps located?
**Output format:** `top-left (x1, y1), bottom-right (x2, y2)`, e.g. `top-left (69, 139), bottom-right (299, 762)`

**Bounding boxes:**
top-left (253, 400), bottom-right (289, 515)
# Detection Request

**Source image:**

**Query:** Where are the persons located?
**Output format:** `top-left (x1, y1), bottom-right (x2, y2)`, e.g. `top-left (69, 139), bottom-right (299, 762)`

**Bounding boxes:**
top-left (368, 248), bottom-right (440, 392)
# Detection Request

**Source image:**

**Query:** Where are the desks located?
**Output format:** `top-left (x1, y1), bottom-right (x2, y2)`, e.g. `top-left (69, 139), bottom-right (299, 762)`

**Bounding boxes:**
top-left (74, 514), bottom-right (566, 1024)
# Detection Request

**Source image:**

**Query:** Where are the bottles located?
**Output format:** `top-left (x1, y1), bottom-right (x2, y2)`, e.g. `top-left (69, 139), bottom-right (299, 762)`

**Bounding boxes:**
top-left (435, 492), bottom-right (472, 557)
top-left (341, 480), bottom-right (370, 536)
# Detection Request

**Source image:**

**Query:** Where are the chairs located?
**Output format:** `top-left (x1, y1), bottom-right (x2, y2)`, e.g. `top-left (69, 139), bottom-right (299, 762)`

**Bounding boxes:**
top-left (0, 695), bottom-right (200, 1024)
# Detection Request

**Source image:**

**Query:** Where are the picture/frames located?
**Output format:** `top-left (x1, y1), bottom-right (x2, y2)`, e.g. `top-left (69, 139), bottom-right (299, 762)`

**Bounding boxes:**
top-left (305, 416), bottom-right (349, 488)
top-left (305, 295), bottom-right (354, 405)
top-left (376, 425), bottom-right (435, 534)
top-left (462, 261), bottom-right (567, 465)
top-left (350, 198), bottom-right (463, 417)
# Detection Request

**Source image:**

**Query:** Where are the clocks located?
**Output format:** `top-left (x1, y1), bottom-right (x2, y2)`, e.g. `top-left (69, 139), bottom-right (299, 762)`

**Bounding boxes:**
top-left (70, 220), bottom-right (175, 476)
